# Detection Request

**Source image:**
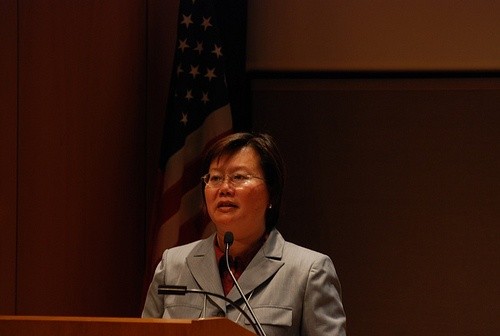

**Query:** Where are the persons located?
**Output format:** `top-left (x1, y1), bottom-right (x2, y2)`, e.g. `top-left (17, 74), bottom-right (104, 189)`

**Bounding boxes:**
top-left (142, 131), bottom-right (346, 336)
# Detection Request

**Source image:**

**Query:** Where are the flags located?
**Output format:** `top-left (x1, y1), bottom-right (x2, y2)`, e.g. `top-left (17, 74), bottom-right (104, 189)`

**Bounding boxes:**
top-left (140, 0), bottom-right (253, 315)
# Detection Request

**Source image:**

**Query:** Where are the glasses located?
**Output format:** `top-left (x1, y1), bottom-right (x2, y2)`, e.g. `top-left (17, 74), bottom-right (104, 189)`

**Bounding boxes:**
top-left (201, 173), bottom-right (264, 189)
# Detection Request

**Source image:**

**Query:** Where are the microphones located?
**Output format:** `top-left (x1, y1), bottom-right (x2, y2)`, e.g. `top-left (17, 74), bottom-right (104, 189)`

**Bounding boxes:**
top-left (155, 285), bottom-right (261, 336)
top-left (223, 231), bottom-right (267, 336)
